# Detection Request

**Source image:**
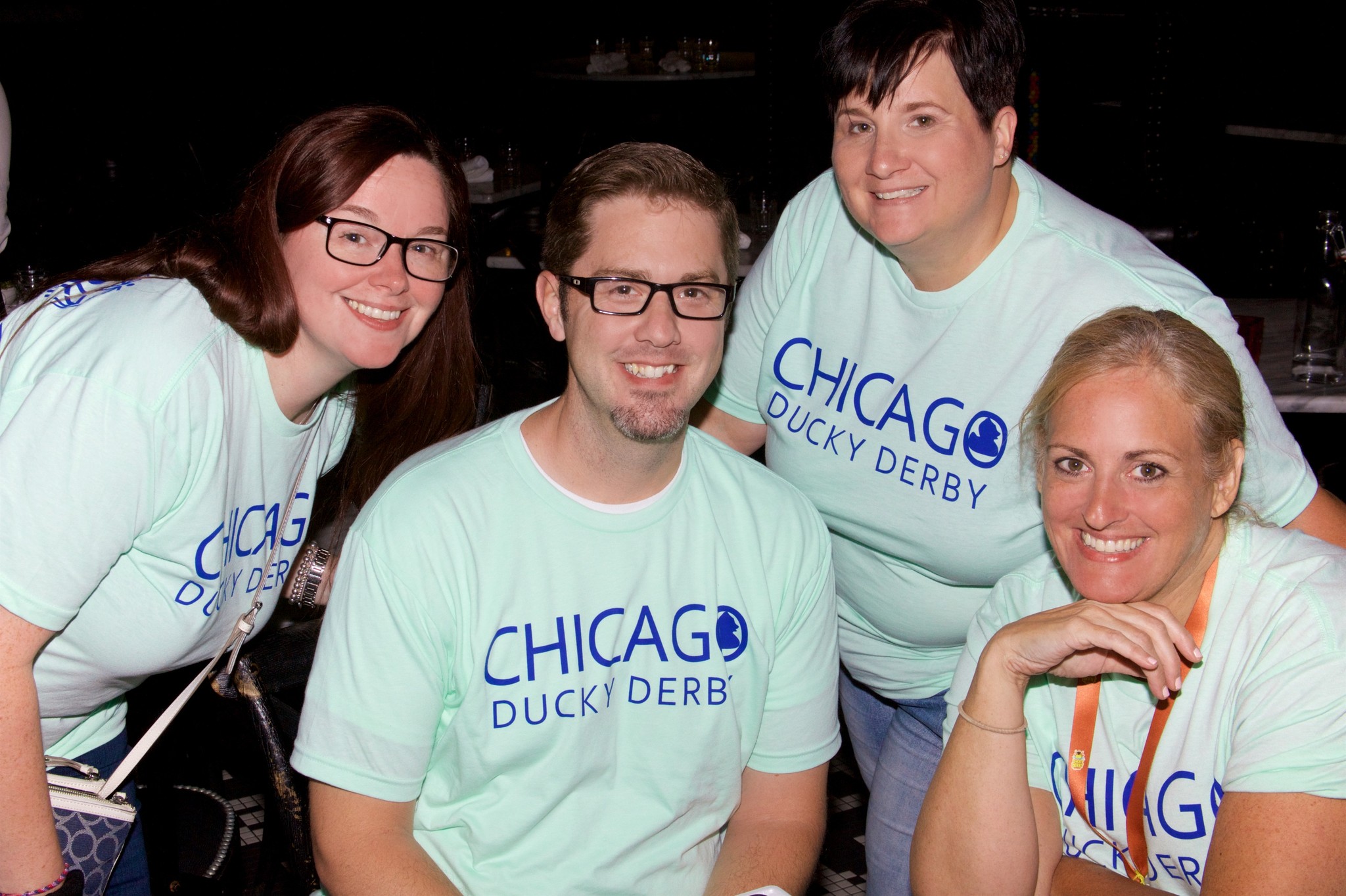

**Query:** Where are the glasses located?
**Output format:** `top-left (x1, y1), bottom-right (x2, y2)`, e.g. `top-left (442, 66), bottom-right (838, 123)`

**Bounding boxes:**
top-left (315, 214), bottom-right (462, 283)
top-left (560, 274), bottom-right (737, 320)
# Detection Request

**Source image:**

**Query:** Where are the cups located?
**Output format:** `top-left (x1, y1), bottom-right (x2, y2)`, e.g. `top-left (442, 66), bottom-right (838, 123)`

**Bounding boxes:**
top-left (748, 189), bottom-right (777, 254)
top-left (590, 37), bottom-right (720, 73)
top-left (455, 137), bottom-right (476, 162)
top-left (1232, 314), bottom-right (1264, 366)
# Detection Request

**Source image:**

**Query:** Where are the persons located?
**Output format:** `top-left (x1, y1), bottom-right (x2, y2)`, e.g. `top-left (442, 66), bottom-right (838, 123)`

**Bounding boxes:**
top-left (294, 139), bottom-right (843, 896)
top-left (692, 1), bottom-right (1345, 896)
top-left (0, 106), bottom-right (481, 896)
top-left (909, 306), bottom-right (1344, 895)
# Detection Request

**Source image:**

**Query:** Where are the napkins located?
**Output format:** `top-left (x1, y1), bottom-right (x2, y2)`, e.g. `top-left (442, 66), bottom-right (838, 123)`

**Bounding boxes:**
top-left (1232, 315), bottom-right (1265, 365)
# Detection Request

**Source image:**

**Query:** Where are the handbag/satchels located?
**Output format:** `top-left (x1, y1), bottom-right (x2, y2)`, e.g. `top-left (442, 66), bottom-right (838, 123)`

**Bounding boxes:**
top-left (39, 755), bottom-right (137, 896)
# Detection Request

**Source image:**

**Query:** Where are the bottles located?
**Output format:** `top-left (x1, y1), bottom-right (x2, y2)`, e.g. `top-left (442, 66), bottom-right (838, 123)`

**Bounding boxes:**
top-left (1290, 211), bottom-right (1346, 385)
top-left (498, 141), bottom-right (522, 191)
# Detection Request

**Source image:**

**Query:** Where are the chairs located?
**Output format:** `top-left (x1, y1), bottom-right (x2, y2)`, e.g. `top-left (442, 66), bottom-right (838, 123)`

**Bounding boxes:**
top-left (208, 619), bottom-right (322, 892)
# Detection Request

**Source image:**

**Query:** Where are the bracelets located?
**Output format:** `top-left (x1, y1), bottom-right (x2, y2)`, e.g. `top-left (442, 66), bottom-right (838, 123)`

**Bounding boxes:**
top-left (734, 885), bottom-right (791, 896)
top-left (1, 861), bottom-right (69, 896)
top-left (46, 869), bottom-right (84, 896)
top-left (958, 699), bottom-right (1028, 735)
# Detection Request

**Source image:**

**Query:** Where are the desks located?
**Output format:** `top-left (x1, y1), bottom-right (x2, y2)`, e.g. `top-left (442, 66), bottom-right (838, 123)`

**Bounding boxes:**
top-left (1226, 298), bottom-right (1346, 499)
